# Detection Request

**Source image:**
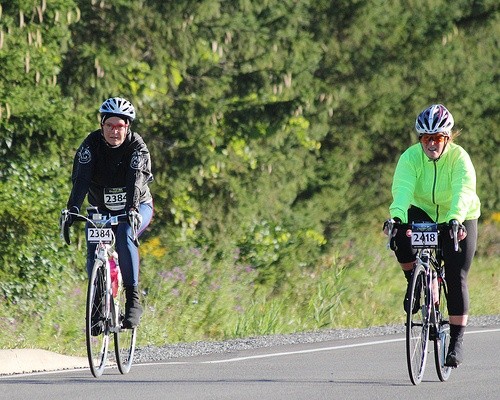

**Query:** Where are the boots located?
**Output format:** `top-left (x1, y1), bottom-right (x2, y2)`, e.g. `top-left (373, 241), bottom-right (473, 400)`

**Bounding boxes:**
top-left (121, 287), bottom-right (144, 328)
top-left (91, 294), bottom-right (104, 336)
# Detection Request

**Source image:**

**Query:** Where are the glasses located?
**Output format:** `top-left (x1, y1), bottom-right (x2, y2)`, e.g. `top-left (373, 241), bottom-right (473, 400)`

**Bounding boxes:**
top-left (102, 123), bottom-right (126, 131)
top-left (421, 133), bottom-right (443, 144)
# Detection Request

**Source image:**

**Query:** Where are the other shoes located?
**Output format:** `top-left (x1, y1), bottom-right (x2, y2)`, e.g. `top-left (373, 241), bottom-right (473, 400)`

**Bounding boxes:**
top-left (404, 275), bottom-right (422, 315)
top-left (445, 339), bottom-right (465, 366)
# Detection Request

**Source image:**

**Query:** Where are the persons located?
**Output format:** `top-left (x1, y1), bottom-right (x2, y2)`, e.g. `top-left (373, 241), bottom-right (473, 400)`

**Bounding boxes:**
top-left (66, 98), bottom-right (155, 337)
top-left (389, 104), bottom-right (481, 369)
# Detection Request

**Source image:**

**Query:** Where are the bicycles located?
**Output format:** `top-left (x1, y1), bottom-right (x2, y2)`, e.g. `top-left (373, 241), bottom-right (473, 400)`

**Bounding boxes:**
top-left (60, 208), bottom-right (138, 377)
top-left (384, 221), bottom-right (465, 385)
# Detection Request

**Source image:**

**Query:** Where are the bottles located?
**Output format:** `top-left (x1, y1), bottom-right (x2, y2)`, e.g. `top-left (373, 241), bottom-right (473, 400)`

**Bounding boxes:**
top-left (429, 269), bottom-right (440, 306)
top-left (109, 256), bottom-right (118, 298)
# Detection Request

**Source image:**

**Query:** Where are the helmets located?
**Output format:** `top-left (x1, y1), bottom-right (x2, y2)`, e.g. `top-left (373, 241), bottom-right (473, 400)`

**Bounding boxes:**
top-left (415, 104), bottom-right (455, 134)
top-left (98, 96), bottom-right (136, 120)
